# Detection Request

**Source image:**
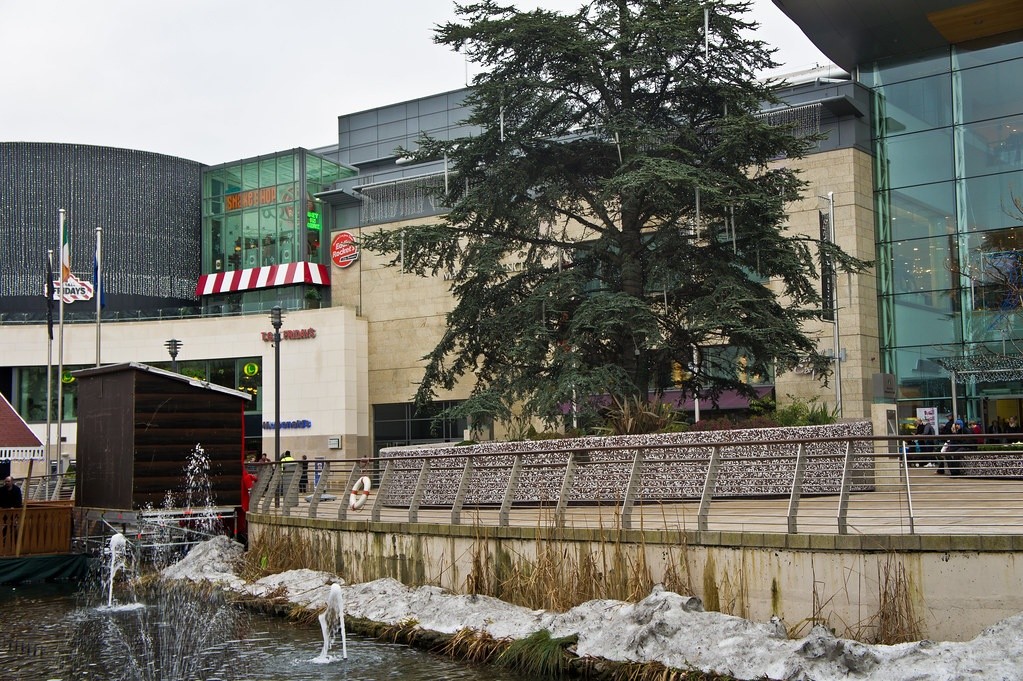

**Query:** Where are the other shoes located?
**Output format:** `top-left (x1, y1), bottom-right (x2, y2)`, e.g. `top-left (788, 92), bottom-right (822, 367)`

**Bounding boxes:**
top-left (933, 463), bottom-right (935, 467)
top-left (915, 463), bottom-right (919, 467)
top-left (934, 472), bottom-right (940, 474)
top-left (923, 462), bottom-right (932, 467)
top-left (911, 463), bottom-right (914, 467)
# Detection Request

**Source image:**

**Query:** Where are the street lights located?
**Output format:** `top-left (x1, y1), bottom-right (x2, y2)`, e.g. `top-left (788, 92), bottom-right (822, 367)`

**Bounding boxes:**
top-left (269, 306), bottom-right (286, 508)
top-left (164, 339), bottom-right (183, 372)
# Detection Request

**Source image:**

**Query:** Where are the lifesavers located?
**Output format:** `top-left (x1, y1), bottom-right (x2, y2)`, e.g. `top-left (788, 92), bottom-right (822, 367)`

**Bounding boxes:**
top-left (349, 476), bottom-right (371, 511)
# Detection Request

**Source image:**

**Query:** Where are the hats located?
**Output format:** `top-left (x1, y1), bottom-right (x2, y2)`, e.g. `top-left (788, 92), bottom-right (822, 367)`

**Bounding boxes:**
top-left (285, 451), bottom-right (290, 455)
top-left (945, 413), bottom-right (952, 416)
top-left (968, 420), bottom-right (975, 424)
top-left (956, 420), bottom-right (964, 429)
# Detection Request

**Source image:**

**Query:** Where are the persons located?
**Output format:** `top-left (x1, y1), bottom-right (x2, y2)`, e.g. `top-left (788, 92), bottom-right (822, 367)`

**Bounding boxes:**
top-left (299, 455), bottom-right (308, 495)
top-left (915, 413), bottom-right (1018, 479)
top-left (0, 476), bottom-right (23, 546)
top-left (281, 451), bottom-right (295, 476)
top-left (247, 453), bottom-right (272, 473)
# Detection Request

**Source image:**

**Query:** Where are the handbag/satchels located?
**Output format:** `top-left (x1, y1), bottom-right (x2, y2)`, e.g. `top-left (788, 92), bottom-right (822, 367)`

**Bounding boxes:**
top-left (941, 442), bottom-right (948, 452)
